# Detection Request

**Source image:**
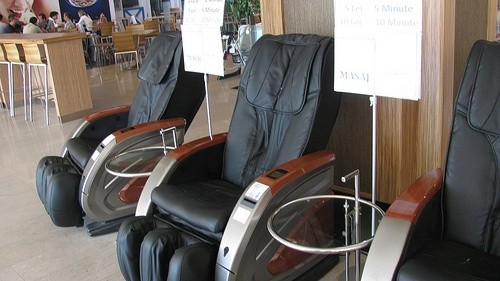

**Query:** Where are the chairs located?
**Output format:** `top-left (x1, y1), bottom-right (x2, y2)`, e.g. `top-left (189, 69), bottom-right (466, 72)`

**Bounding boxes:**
top-left (360, 40), bottom-right (500, 281)
top-left (58, 8), bottom-right (183, 71)
top-left (36, 32), bottom-right (210, 237)
top-left (116, 34), bottom-right (343, 281)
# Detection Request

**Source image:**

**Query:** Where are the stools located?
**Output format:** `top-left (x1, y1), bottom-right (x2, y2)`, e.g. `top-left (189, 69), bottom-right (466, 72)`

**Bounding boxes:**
top-left (0, 43), bottom-right (54, 126)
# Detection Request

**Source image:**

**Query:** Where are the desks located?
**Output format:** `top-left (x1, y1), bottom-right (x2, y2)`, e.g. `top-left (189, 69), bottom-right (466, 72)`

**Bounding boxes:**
top-left (267, 195), bottom-right (384, 281)
top-left (104, 146), bottom-right (176, 179)
top-left (0, 33), bottom-right (94, 124)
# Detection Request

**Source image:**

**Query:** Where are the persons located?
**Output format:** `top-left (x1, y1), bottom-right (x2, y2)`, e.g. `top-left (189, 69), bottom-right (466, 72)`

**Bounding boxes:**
top-left (23, 17), bottom-right (42, 34)
top-left (3, 13), bottom-right (24, 33)
top-left (78, 10), bottom-right (93, 33)
top-left (38, 14), bottom-right (50, 33)
top-left (96, 13), bottom-right (107, 36)
top-left (48, 11), bottom-right (58, 30)
top-left (0, 14), bottom-right (11, 33)
top-left (63, 12), bottom-right (76, 29)
